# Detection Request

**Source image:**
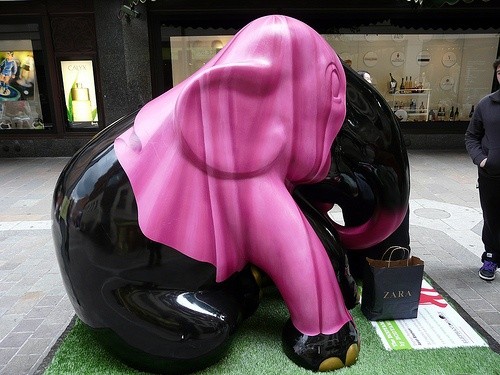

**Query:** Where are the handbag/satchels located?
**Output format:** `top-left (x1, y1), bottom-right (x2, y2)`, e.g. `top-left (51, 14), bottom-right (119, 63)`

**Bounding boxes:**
top-left (361, 246), bottom-right (424, 321)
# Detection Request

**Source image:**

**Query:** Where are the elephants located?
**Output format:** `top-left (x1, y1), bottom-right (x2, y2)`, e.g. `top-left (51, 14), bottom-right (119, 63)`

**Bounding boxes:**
top-left (51, 15), bottom-right (410, 372)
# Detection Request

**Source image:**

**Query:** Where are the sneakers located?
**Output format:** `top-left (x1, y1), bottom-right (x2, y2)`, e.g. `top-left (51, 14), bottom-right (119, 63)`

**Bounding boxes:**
top-left (478, 260), bottom-right (497, 280)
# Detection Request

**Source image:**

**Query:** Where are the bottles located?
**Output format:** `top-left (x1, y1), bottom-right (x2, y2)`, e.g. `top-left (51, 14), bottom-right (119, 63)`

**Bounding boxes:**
top-left (413, 81), bottom-right (423, 93)
top-left (455, 107), bottom-right (460, 121)
top-left (409, 99), bottom-right (416, 113)
top-left (400, 76), bottom-right (412, 93)
top-left (437, 106), bottom-right (445, 121)
top-left (429, 108), bottom-right (435, 121)
top-left (399, 101), bottom-right (404, 109)
top-left (394, 101), bottom-right (398, 109)
top-left (450, 106), bottom-right (454, 121)
top-left (419, 101), bottom-right (425, 113)
top-left (469, 105), bottom-right (475, 120)
top-left (389, 73), bottom-right (397, 93)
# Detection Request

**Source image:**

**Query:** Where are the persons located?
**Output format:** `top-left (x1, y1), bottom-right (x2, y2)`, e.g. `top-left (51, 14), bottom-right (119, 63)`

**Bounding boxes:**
top-left (465, 59), bottom-right (500, 279)
top-left (0, 51), bottom-right (17, 95)
